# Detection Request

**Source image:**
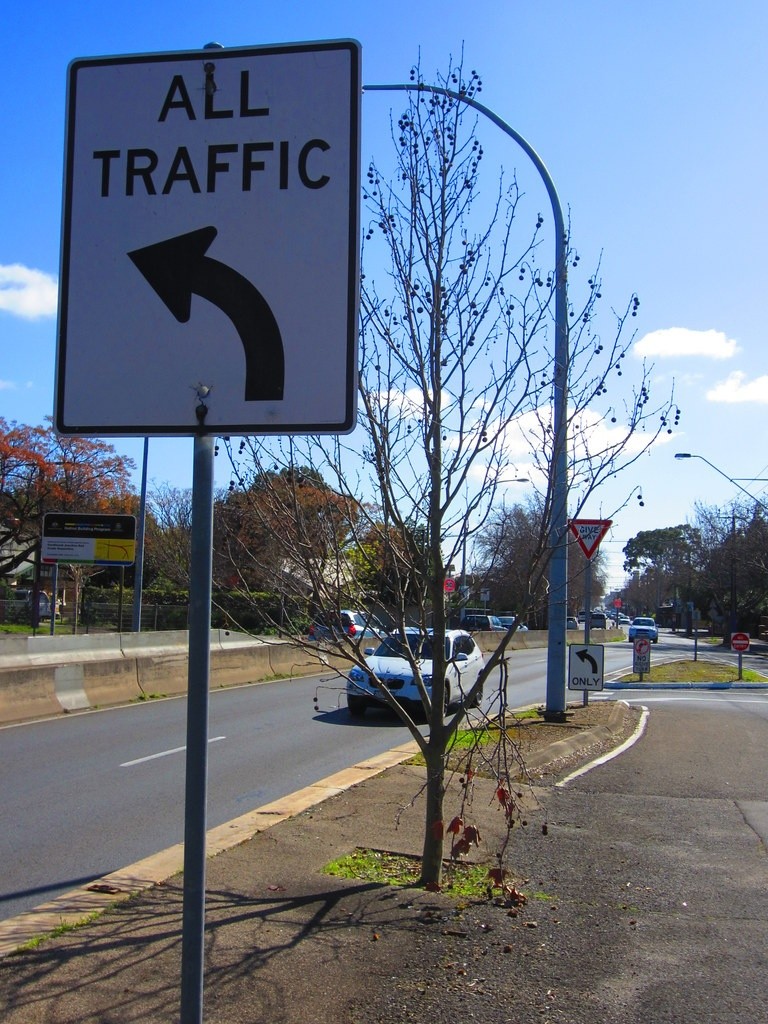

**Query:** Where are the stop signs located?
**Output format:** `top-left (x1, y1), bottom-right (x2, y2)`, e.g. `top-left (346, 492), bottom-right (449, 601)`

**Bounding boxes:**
top-left (730, 633), bottom-right (750, 652)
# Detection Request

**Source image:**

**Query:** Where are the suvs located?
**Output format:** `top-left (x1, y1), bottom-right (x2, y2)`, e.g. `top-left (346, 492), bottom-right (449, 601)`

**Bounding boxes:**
top-left (308, 610), bottom-right (382, 640)
top-left (567, 616), bottom-right (579, 629)
top-left (346, 624), bottom-right (486, 719)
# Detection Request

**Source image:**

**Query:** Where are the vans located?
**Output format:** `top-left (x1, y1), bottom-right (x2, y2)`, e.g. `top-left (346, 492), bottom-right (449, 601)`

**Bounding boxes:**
top-left (577, 611), bottom-right (589, 623)
top-left (590, 613), bottom-right (606, 630)
top-left (13, 590), bottom-right (49, 621)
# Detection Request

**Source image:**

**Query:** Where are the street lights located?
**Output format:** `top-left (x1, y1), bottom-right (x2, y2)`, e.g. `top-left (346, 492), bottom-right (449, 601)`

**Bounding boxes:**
top-left (459, 477), bottom-right (530, 618)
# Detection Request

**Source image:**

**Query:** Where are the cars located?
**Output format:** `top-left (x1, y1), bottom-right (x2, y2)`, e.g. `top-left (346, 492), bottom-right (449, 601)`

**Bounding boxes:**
top-left (498, 616), bottom-right (528, 631)
top-left (629, 617), bottom-right (658, 643)
top-left (460, 616), bottom-right (509, 631)
top-left (602, 609), bottom-right (630, 624)
